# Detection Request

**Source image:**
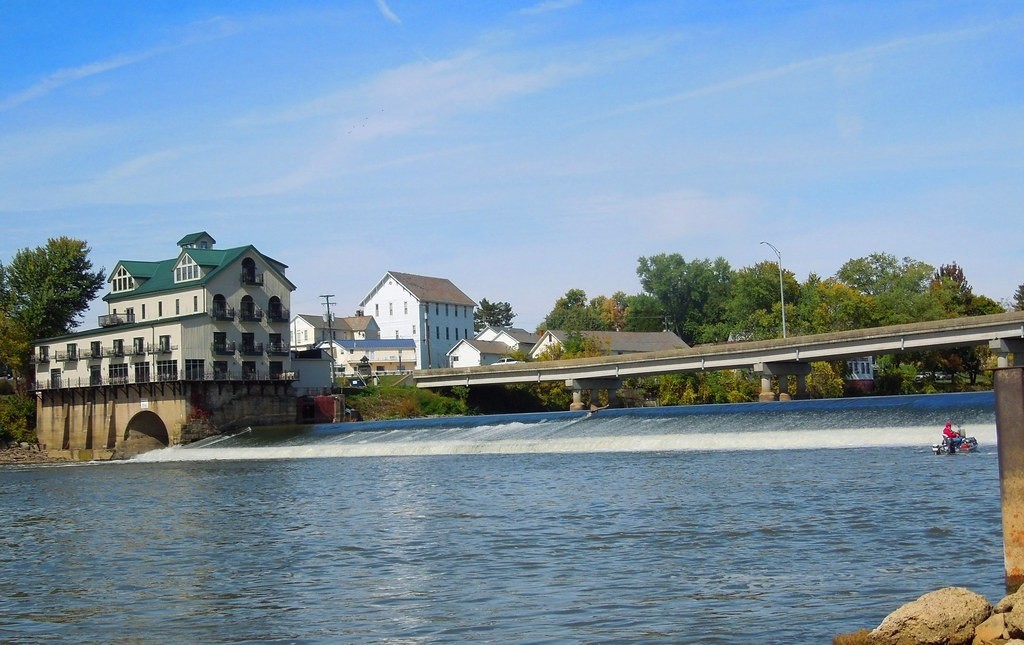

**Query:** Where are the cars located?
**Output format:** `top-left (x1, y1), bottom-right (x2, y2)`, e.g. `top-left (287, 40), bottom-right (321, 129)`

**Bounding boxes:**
top-left (915, 370), bottom-right (959, 382)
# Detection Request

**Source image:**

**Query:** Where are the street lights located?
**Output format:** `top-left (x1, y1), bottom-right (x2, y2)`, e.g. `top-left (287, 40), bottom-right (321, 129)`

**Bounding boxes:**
top-left (759, 241), bottom-right (786, 338)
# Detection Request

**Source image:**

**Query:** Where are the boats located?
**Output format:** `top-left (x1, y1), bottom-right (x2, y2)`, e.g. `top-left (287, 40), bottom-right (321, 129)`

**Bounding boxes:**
top-left (932, 427), bottom-right (981, 457)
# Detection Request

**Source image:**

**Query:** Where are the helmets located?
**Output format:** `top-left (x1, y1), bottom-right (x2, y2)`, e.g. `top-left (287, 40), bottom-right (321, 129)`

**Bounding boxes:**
top-left (946, 422), bottom-right (951, 426)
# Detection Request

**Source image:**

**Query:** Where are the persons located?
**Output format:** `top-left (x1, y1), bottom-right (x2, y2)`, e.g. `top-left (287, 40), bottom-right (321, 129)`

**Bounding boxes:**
top-left (944, 422), bottom-right (962, 449)
